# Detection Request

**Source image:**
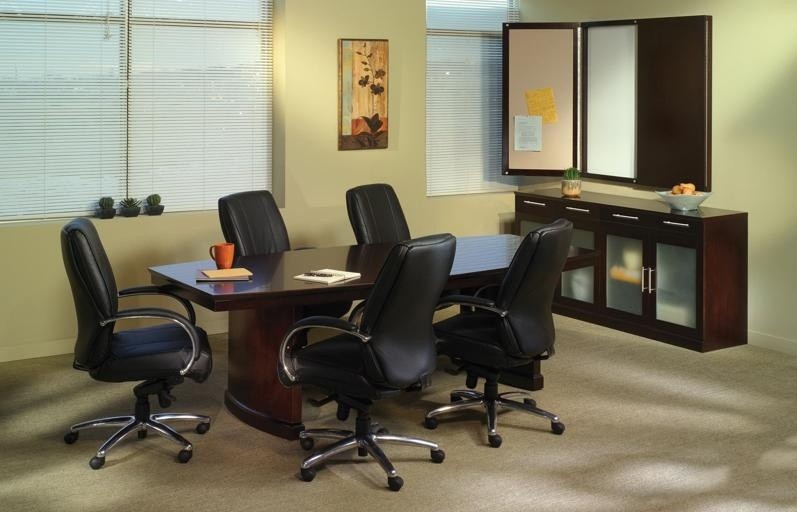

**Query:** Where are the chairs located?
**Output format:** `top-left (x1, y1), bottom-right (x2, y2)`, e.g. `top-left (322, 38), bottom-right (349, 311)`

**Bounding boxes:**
top-left (61, 217), bottom-right (212, 470)
top-left (425, 217), bottom-right (575, 453)
top-left (276, 233), bottom-right (456, 493)
top-left (346, 183), bottom-right (412, 247)
top-left (218, 190), bottom-right (352, 345)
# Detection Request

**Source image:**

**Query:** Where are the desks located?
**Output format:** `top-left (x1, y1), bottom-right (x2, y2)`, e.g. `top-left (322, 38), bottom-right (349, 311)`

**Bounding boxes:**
top-left (148, 231), bottom-right (596, 442)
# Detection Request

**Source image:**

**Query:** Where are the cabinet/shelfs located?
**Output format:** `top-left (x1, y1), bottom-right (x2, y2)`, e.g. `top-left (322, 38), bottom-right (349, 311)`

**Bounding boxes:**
top-left (602, 198), bottom-right (748, 353)
top-left (502, 15), bottom-right (715, 192)
top-left (514, 185), bottom-right (603, 328)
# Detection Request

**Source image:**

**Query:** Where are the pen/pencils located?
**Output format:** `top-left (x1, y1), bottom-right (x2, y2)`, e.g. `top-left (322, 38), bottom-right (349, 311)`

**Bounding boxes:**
top-left (304, 272), bottom-right (333, 277)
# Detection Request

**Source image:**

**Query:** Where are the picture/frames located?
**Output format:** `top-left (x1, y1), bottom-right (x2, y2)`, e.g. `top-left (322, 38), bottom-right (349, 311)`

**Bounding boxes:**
top-left (337, 36), bottom-right (390, 151)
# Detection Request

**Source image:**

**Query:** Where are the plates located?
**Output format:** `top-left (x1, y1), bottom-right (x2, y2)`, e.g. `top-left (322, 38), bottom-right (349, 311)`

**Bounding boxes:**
top-left (655, 189), bottom-right (713, 212)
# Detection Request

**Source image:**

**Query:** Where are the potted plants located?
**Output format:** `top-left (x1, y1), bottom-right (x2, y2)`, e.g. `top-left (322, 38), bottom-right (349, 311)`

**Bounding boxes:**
top-left (119, 196), bottom-right (143, 216)
top-left (560, 166), bottom-right (582, 200)
top-left (144, 194), bottom-right (165, 215)
top-left (95, 198), bottom-right (116, 219)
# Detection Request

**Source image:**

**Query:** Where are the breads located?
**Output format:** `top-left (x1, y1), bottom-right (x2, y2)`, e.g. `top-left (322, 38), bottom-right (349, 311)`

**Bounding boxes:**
top-left (673, 183), bottom-right (704, 196)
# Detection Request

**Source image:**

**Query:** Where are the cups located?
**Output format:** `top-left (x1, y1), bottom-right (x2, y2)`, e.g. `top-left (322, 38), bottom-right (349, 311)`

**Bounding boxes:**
top-left (210, 242), bottom-right (236, 269)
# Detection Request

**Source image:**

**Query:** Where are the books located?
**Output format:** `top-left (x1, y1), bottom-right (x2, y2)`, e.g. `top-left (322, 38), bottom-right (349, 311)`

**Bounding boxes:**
top-left (293, 267), bottom-right (361, 285)
top-left (200, 267), bottom-right (254, 280)
